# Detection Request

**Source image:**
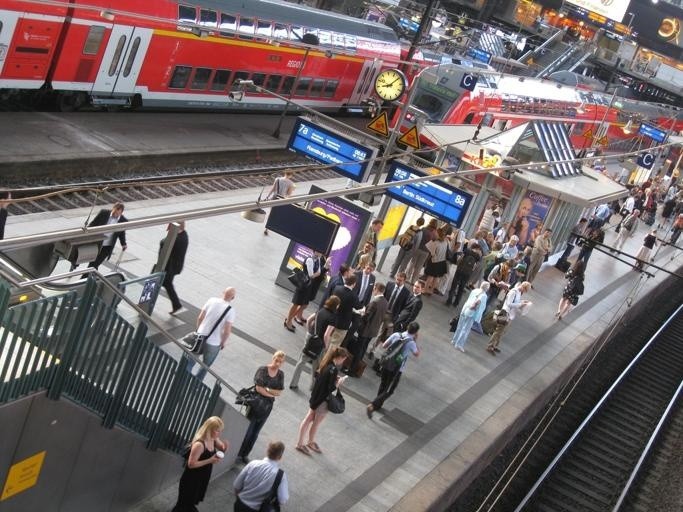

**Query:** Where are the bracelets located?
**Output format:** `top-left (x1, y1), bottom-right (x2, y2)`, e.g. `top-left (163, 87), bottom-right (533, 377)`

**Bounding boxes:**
top-left (266, 387), bottom-right (269, 392)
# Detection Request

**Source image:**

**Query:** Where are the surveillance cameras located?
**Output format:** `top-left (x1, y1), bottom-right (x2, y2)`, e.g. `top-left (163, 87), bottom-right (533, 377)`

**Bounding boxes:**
top-left (229, 91), bottom-right (244, 102)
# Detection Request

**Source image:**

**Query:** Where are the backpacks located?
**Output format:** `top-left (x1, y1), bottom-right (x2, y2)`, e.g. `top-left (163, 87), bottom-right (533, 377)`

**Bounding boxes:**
top-left (378, 332), bottom-right (415, 373)
top-left (459, 250), bottom-right (477, 275)
top-left (399, 226), bottom-right (421, 250)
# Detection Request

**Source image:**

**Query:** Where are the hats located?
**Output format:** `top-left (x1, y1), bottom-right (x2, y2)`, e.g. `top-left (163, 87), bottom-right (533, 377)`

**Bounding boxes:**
top-left (512, 264), bottom-right (524, 271)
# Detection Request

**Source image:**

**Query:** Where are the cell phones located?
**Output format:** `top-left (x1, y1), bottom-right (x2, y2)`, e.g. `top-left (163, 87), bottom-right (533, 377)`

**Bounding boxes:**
top-left (338, 375), bottom-right (348, 381)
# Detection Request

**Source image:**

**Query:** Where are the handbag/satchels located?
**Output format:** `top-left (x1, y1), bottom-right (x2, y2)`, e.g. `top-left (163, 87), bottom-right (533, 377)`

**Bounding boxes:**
top-left (235, 387), bottom-right (263, 406)
top-left (178, 331), bottom-right (205, 355)
top-left (327, 389), bottom-right (345, 413)
top-left (260, 491), bottom-right (280, 511)
top-left (545, 255), bottom-right (548, 261)
top-left (492, 310), bottom-right (510, 326)
top-left (446, 249), bottom-right (453, 260)
top-left (180, 443), bottom-right (191, 457)
top-left (303, 335), bottom-right (324, 360)
top-left (349, 362), bottom-right (366, 378)
top-left (288, 270), bottom-right (311, 290)
top-left (449, 315), bottom-right (459, 332)
top-left (484, 263), bottom-right (501, 281)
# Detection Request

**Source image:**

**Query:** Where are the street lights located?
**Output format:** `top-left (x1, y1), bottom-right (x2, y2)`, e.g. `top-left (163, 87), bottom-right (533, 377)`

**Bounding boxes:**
top-left (615, 11), bottom-right (637, 54)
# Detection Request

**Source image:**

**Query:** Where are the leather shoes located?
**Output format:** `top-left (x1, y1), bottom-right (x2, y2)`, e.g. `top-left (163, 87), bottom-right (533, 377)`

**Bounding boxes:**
top-left (284, 319), bottom-right (295, 331)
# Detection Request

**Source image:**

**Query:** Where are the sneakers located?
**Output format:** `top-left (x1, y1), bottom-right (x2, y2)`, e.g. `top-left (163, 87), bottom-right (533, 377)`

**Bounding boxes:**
top-left (296, 447), bottom-right (310, 455)
top-left (308, 442), bottom-right (321, 453)
top-left (294, 316), bottom-right (307, 325)
top-left (367, 405), bottom-right (372, 419)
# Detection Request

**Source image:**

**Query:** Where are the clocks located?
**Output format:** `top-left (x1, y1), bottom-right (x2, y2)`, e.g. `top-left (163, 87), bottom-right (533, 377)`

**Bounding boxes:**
top-left (371, 66), bottom-right (409, 104)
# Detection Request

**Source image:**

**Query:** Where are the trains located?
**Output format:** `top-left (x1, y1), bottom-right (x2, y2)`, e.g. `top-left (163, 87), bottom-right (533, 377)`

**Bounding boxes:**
top-left (391, 63), bottom-right (683, 165)
top-left (0, 0), bottom-right (496, 117)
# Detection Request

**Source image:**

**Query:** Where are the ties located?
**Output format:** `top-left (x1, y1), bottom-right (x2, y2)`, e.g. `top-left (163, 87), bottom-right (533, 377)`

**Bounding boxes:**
top-left (358, 275), bottom-right (367, 300)
top-left (388, 287), bottom-right (398, 309)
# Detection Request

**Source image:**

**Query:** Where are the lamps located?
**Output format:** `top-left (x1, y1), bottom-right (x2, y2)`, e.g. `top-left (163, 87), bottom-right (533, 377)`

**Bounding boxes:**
top-left (240, 208), bottom-right (266, 223)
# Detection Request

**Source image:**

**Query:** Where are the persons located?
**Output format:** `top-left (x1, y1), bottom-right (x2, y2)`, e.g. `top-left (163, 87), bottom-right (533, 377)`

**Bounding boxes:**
top-left (232, 441), bottom-right (289, 512)
top-left (236, 350), bottom-right (286, 464)
top-left (81, 202), bottom-right (128, 279)
top-left (261, 168), bottom-right (296, 235)
top-left (0, 192), bottom-right (11, 241)
top-left (186, 288), bottom-right (237, 381)
top-left (171, 416), bottom-right (228, 512)
top-left (151, 220), bottom-right (189, 314)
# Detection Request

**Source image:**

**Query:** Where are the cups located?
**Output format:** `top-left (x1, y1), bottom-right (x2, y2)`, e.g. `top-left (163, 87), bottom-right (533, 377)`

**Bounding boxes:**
top-left (215, 451), bottom-right (224, 459)
